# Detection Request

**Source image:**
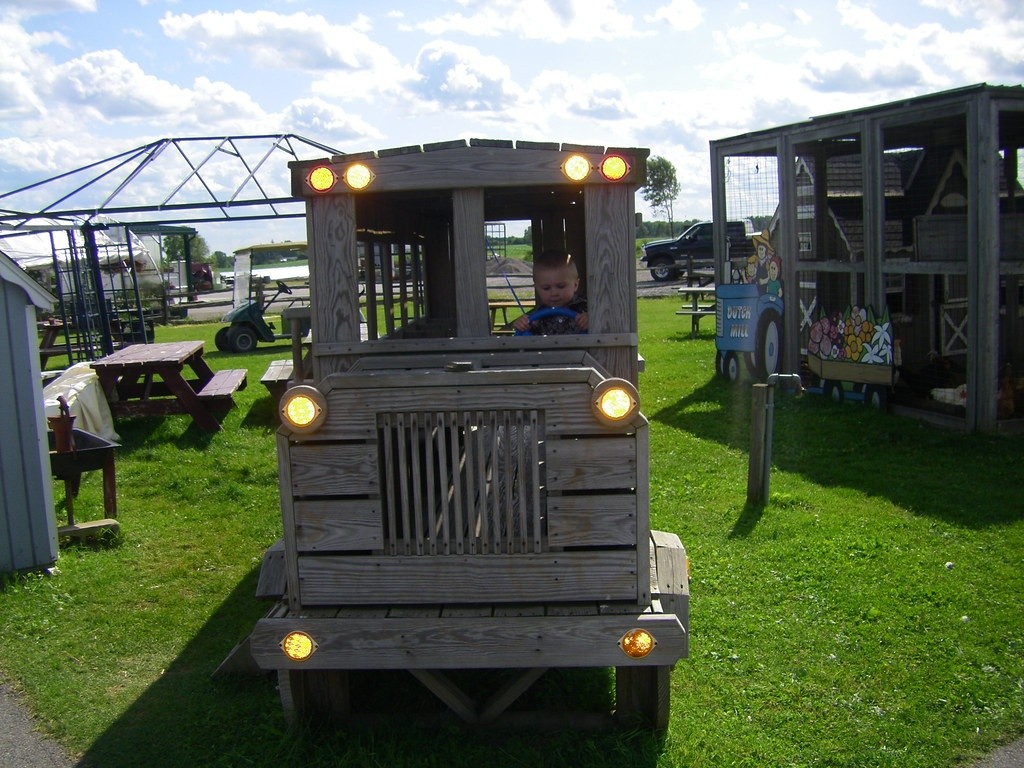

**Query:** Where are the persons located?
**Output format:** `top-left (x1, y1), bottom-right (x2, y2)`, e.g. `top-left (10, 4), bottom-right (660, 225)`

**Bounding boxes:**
top-left (511, 248), bottom-right (588, 334)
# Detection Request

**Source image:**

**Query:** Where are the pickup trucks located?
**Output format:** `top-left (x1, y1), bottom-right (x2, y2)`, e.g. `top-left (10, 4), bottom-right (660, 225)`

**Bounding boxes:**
top-left (640, 218), bottom-right (774, 282)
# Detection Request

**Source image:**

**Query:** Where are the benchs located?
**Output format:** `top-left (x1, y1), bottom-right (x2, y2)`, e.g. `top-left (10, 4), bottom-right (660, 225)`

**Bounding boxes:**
top-left (492, 331), bottom-right (516, 334)
top-left (264, 323), bottom-right (380, 402)
top-left (676, 311), bottom-right (715, 315)
top-left (682, 305), bottom-right (711, 309)
top-left (197, 368), bottom-right (248, 401)
top-left (39, 342), bottom-right (127, 354)
top-left (260, 360), bottom-right (294, 384)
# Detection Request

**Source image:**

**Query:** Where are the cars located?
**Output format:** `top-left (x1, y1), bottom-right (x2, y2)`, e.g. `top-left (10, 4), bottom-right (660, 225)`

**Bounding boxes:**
top-left (210, 139), bottom-right (691, 737)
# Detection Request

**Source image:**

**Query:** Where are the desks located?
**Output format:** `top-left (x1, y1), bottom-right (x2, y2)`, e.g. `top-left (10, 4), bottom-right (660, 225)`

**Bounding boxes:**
top-left (488, 301), bottom-right (536, 331)
top-left (39, 319), bottom-right (122, 372)
top-left (682, 271), bottom-right (714, 300)
top-left (678, 287), bottom-right (716, 338)
top-left (494, 323), bottom-right (505, 326)
top-left (90, 341), bottom-right (239, 434)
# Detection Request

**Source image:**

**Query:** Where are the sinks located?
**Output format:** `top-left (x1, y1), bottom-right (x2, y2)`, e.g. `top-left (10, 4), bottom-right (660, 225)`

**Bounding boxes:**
top-left (46, 427), bottom-right (123, 476)
